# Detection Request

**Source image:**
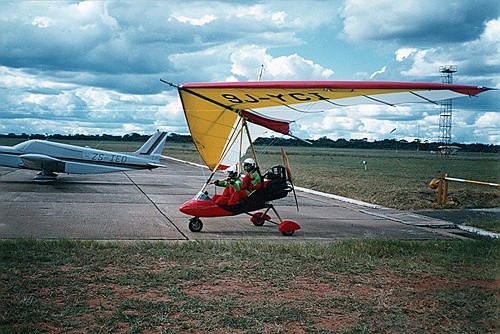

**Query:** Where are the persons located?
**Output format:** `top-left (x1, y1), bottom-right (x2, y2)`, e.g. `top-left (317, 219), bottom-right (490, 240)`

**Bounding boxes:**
top-left (209, 162), bottom-right (241, 205)
top-left (230, 158), bottom-right (261, 205)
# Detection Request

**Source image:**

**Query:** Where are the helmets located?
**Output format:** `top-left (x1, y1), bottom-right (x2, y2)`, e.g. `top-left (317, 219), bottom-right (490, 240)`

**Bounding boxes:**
top-left (241, 158), bottom-right (257, 171)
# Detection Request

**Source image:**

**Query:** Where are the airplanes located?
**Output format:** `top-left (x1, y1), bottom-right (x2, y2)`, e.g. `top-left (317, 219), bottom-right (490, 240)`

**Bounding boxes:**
top-left (0, 125), bottom-right (173, 184)
top-left (157, 64), bottom-right (500, 236)
